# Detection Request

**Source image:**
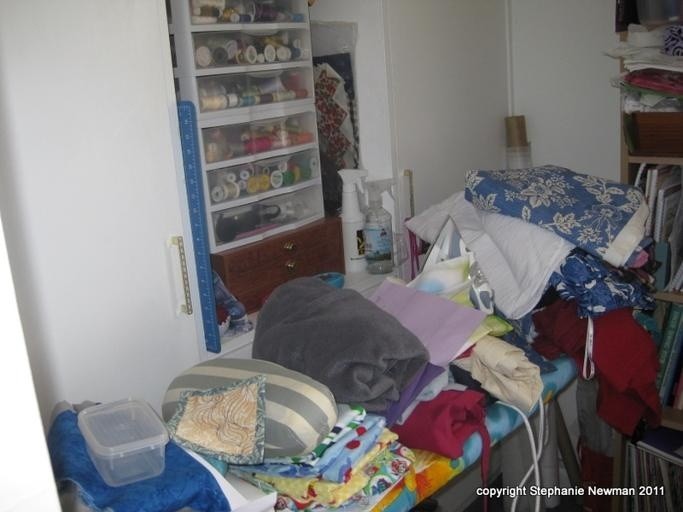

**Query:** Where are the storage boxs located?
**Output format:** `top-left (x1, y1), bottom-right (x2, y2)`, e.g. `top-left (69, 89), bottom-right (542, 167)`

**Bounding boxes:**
top-left (78, 398), bottom-right (172, 488)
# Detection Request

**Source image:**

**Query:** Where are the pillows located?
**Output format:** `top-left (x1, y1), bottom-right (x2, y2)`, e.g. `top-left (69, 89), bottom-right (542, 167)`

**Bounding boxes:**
top-left (161, 357), bottom-right (338, 457)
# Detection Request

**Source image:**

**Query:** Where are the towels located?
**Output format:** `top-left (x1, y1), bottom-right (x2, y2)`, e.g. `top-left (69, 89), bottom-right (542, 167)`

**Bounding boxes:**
top-left (47, 410), bottom-right (231, 511)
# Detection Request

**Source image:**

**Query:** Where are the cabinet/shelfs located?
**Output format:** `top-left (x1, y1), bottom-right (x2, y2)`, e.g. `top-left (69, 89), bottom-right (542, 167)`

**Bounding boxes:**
top-left (166, 0), bottom-right (326, 255)
top-left (208, 216), bottom-right (345, 325)
top-left (611, 2), bottom-right (683, 512)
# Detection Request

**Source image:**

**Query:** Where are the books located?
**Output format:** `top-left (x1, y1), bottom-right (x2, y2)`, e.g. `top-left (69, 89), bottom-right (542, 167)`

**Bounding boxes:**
top-left (620, 426), bottom-right (683, 512)
top-left (628, 161), bottom-right (683, 292)
top-left (641, 299), bottom-right (683, 410)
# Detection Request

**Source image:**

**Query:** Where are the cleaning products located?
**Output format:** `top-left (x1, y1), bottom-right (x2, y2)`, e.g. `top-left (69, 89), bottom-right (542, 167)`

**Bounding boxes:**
top-left (361, 178), bottom-right (399, 275)
top-left (337, 169), bottom-right (369, 274)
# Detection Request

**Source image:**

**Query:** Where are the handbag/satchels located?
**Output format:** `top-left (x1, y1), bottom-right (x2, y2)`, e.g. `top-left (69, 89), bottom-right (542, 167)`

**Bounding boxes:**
top-left (217, 204), bottom-right (281, 234)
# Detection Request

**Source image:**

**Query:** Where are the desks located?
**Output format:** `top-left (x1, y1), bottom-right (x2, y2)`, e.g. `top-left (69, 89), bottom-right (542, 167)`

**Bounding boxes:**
top-left (292, 354), bottom-right (589, 512)
